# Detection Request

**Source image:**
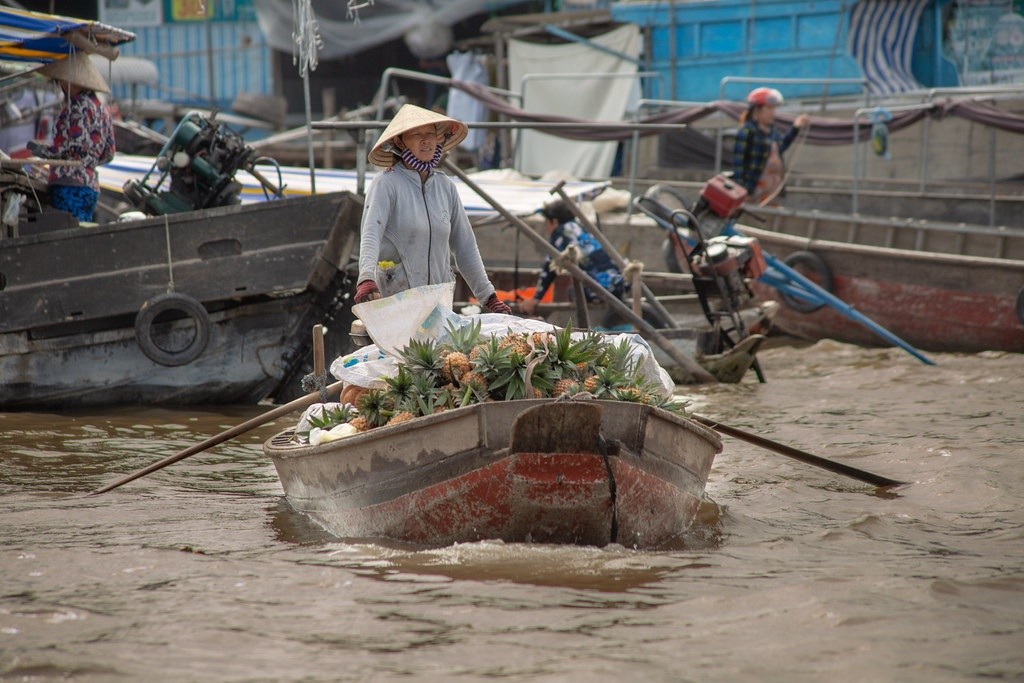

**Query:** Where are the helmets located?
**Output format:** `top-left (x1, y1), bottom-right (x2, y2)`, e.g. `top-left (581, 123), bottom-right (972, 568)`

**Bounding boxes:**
top-left (748, 88), bottom-right (785, 107)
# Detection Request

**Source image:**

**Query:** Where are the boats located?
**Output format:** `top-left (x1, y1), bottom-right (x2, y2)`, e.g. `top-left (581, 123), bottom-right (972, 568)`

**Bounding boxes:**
top-left (0, 6), bottom-right (366, 409)
top-left (82, 320), bottom-right (906, 544)
top-left (92, 153), bottom-right (1024, 384)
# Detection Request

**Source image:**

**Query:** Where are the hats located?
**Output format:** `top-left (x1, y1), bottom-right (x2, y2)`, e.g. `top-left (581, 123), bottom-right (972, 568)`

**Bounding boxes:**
top-left (368, 103), bottom-right (468, 167)
top-left (37, 51), bottom-right (111, 93)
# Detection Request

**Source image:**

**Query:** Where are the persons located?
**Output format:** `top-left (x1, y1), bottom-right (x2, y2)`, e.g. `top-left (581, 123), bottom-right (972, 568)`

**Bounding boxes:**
top-left (354, 103), bottom-right (512, 316)
top-left (519, 202), bottom-right (631, 315)
top-left (730, 87), bottom-right (810, 209)
top-left (33, 51), bottom-right (116, 223)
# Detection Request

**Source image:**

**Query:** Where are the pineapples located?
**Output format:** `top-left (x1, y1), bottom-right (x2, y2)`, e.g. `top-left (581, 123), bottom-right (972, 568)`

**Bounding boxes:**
top-left (306, 318), bottom-right (693, 431)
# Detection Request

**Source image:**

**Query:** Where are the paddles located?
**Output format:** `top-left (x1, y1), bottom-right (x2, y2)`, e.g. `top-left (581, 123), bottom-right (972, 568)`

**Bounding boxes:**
top-left (82, 384), bottom-right (342, 498)
top-left (695, 414), bottom-right (904, 490)
top-left (436, 150), bottom-right (720, 384)
top-left (549, 179), bottom-right (683, 329)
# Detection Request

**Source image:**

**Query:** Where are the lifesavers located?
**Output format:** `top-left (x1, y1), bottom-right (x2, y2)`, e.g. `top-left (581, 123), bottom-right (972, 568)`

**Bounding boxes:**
top-left (133, 292), bottom-right (212, 365)
top-left (777, 250), bottom-right (834, 313)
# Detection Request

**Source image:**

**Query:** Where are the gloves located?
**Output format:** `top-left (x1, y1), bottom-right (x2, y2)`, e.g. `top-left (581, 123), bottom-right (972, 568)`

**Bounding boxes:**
top-left (354, 281), bottom-right (381, 304)
top-left (485, 294), bottom-right (513, 315)
